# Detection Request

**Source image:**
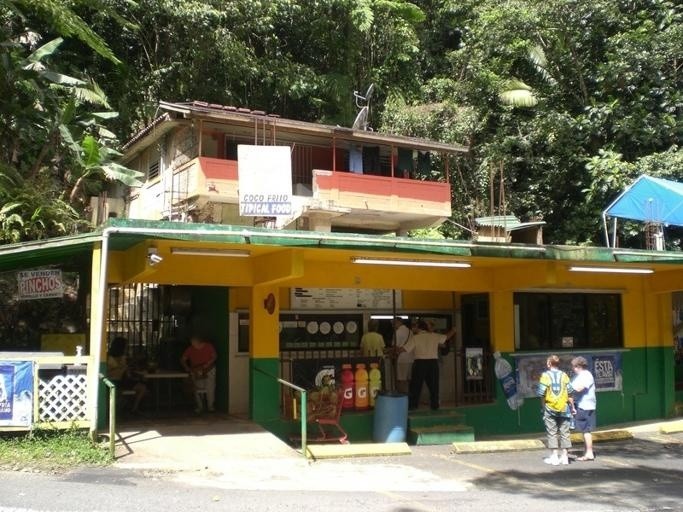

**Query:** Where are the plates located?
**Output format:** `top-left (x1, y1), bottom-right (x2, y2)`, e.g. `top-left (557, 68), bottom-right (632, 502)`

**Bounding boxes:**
top-left (319, 321), bottom-right (331, 334)
top-left (332, 321), bottom-right (344, 334)
top-left (347, 321), bottom-right (356, 334)
top-left (305, 321), bottom-right (318, 334)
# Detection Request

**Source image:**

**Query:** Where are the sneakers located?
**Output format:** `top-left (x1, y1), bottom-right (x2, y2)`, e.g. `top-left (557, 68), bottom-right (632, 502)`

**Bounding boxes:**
top-left (577, 454), bottom-right (595, 461)
top-left (559, 456), bottom-right (568, 464)
top-left (545, 458), bottom-right (559, 465)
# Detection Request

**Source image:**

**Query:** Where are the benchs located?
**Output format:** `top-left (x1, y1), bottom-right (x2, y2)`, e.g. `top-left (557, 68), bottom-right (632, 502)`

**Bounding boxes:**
top-left (120, 389), bottom-right (137, 420)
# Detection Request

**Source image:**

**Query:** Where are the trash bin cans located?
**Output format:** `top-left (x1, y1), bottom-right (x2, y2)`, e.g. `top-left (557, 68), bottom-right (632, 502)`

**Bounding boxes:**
top-left (373, 391), bottom-right (409, 443)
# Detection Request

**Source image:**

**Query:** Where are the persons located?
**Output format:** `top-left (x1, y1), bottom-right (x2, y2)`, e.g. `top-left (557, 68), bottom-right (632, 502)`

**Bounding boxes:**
top-left (570, 357), bottom-right (597, 460)
top-left (181, 337), bottom-right (218, 417)
top-left (538, 354), bottom-right (578, 466)
top-left (106, 337), bottom-right (148, 416)
top-left (359, 318), bottom-right (457, 414)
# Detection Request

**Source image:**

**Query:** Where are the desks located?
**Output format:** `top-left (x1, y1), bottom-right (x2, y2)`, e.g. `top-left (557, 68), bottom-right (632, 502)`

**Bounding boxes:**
top-left (142, 367), bottom-right (190, 420)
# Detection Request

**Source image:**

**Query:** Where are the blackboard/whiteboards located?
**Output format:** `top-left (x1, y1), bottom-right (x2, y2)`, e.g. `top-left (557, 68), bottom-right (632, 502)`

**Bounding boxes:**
top-left (513, 292), bottom-right (623, 350)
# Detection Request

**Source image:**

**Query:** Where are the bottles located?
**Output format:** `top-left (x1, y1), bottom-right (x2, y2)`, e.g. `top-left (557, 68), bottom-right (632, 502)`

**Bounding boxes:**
top-left (75, 346), bottom-right (83, 365)
top-left (368, 363), bottom-right (382, 407)
top-left (354, 363), bottom-right (369, 407)
top-left (340, 364), bottom-right (354, 407)
top-left (492, 351), bottom-right (526, 409)
top-left (569, 413), bottom-right (576, 430)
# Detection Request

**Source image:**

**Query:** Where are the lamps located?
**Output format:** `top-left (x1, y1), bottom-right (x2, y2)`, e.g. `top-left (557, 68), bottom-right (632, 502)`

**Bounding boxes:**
top-left (171, 247), bottom-right (251, 258)
top-left (352, 257), bottom-right (472, 268)
top-left (567, 264), bottom-right (654, 274)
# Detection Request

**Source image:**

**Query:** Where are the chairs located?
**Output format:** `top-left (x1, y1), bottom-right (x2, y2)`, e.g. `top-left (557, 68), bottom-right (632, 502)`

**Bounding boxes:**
top-left (287, 392), bottom-right (350, 447)
